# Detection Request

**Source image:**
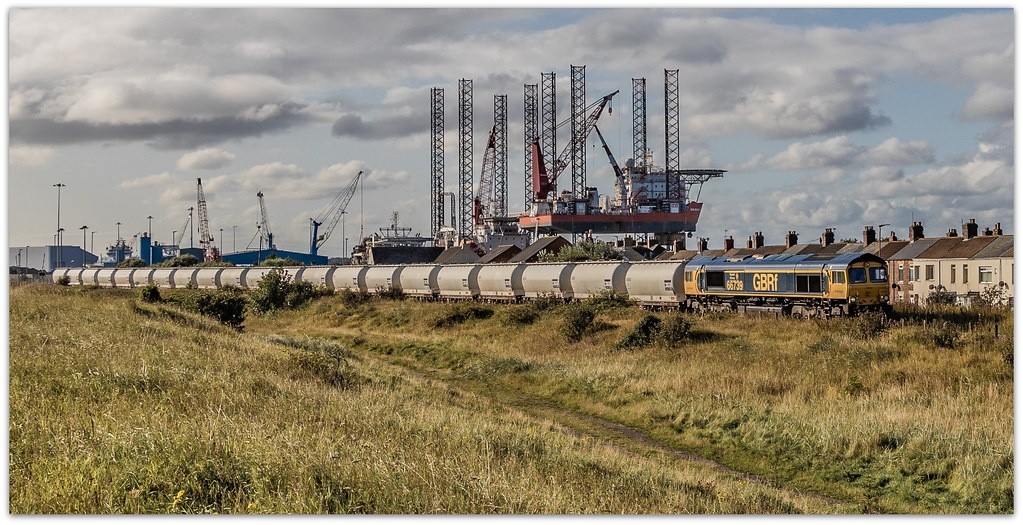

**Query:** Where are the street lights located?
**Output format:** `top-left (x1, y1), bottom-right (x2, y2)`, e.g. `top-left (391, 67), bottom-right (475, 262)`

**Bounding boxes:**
top-left (188, 207), bottom-right (198, 249)
top-left (147, 216), bottom-right (154, 245)
top-left (116, 222), bottom-right (121, 244)
top-left (879, 224), bottom-right (890, 279)
top-left (79, 226), bottom-right (89, 250)
top-left (172, 230), bottom-right (178, 255)
top-left (53, 183), bottom-right (66, 267)
top-left (220, 229), bottom-right (224, 264)
top-left (341, 211), bottom-right (349, 266)
top-left (232, 225), bottom-right (238, 253)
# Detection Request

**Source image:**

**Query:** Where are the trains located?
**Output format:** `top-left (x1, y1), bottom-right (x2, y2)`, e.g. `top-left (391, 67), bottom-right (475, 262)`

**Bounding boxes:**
top-left (53, 253), bottom-right (889, 324)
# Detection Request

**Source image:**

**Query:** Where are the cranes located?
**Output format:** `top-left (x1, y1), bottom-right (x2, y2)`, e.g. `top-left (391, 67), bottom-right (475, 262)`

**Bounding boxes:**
top-left (308, 171), bottom-right (364, 251)
top-left (535, 90), bottom-right (620, 185)
top-left (474, 123), bottom-right (494, 246)
top-left (256, 190), bottom-right (275, 251)
top-left (174, 176), bottom-right (215, 263)
top-left (592, 124), bottom-right (624, 184)
